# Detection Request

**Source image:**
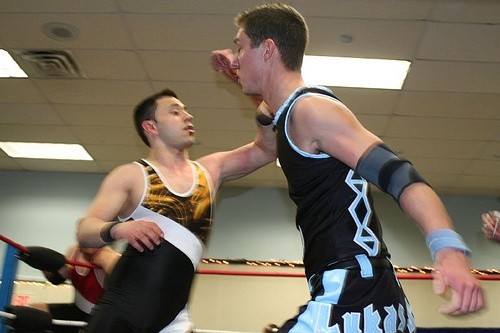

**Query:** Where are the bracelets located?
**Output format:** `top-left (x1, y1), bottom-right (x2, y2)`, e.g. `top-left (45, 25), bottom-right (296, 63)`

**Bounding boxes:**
top-left (426, 229), bottom-right (472, 263)
top-left (100, 221), bottom-right (121, 242)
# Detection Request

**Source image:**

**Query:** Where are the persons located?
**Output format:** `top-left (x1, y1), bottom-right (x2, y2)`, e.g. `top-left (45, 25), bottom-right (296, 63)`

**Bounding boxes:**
top-left (78, 88), bottom-right (277, 332)
top-left (211, 3), bottom-right (484, 333)
top-left (27, 219), bottom-right (123, 324)
top-left (481, 211), bottom-right (500, 243)
